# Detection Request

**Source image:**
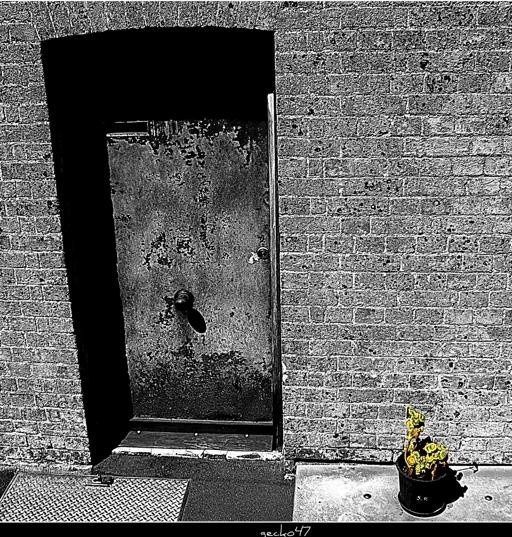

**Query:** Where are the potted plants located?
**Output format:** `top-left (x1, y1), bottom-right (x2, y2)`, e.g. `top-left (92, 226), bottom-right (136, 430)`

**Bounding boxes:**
top-left (396, 405), bottom-right (447, 518)
